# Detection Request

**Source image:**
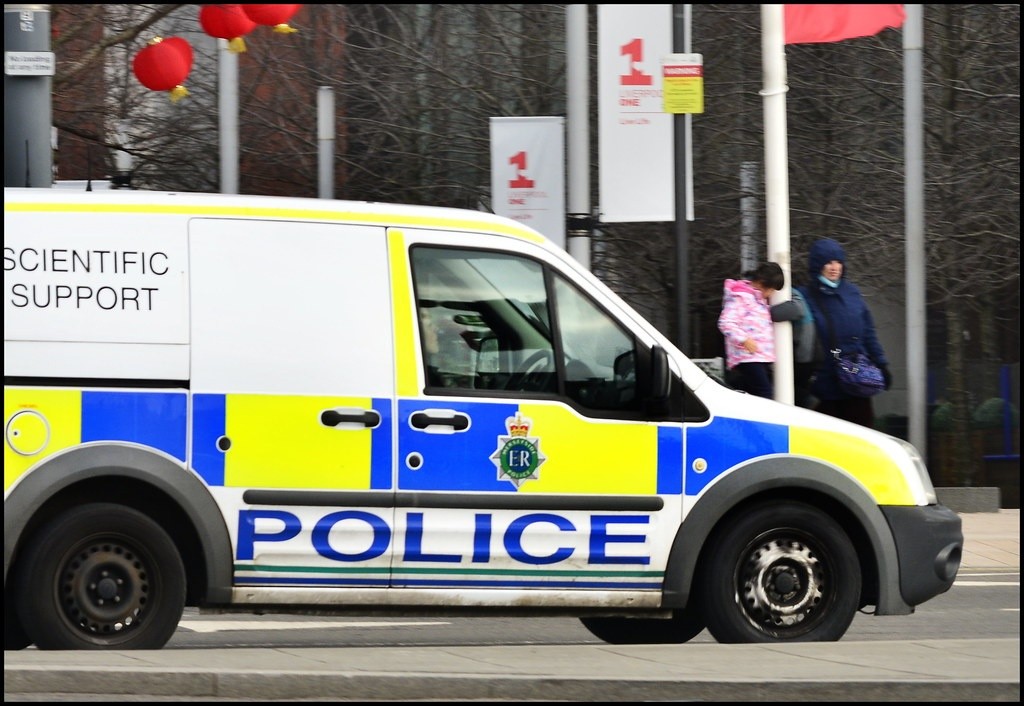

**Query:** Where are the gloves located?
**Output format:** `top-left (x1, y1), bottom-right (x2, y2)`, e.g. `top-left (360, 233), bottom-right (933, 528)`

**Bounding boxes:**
top-left (832, 348), bottom-right (885, 398)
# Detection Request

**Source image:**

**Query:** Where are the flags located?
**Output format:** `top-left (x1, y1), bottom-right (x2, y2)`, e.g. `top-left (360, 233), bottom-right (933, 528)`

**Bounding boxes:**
top-left (784, 4), bottom-right (909, 46)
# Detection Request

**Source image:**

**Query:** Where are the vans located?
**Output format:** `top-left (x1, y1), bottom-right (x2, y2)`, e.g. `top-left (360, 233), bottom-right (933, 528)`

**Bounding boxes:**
top-left (4, 193), bottom-right (964, 650)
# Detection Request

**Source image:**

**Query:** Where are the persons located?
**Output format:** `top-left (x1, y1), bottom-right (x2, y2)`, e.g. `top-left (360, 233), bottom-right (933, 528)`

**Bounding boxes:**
top-left (717, 262), bottom-right (785, 401)
top-left (418, 304), bottom-right (460, 391)
top-left (770, 237), bottom-right (894, 431)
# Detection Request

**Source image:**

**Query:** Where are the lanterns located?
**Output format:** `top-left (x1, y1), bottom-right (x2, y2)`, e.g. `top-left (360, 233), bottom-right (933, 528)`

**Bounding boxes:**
top-left (133, 35), bottom-right (195, 103)
top-left (200, 5), bottom-right (304, 55)
